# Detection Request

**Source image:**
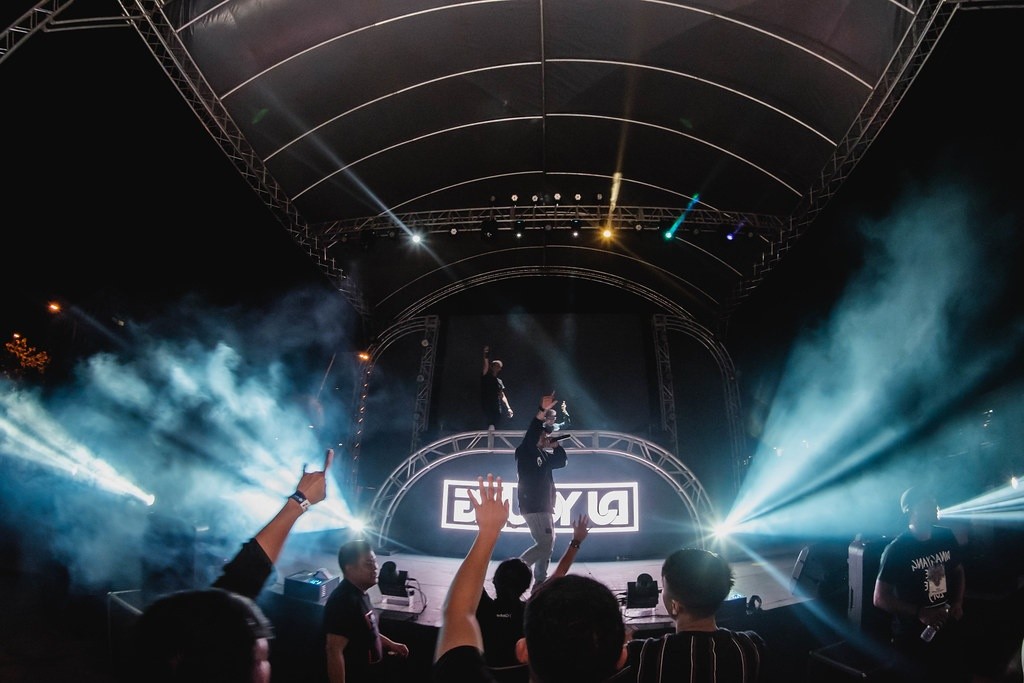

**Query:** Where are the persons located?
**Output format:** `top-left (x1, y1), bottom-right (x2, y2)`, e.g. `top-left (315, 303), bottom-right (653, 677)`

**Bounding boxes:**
top-left (515, 390), bottom-right (568, 591)
top-left (482, 345), bottom-right (514, 430)
top-left (0, 449), bottom-right (966, 683)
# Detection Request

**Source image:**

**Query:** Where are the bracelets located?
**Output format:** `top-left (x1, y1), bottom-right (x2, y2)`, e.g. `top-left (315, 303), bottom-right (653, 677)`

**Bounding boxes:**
top-left (507, 407), bottom-right (511, 409)
top-left (288, 490), bottom-right (311, 513)
top-left (539, 406), bottom-right (548, 412)
top-left (569, 540), bottom-right (580, 548)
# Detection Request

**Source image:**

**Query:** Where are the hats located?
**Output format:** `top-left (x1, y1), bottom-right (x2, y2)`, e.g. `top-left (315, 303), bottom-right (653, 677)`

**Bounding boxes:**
top-left (544, 409), bottom-right (557, 417)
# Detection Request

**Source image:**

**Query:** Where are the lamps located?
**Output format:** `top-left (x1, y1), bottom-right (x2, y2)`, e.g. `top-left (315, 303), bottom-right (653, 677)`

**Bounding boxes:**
top-left (412, 411), bottom-right (423, 421)
top-left (449, 225), bottom-right (459, 235)
top-left (659, 333), bottom-right (667, 343)
top-left (664, 372), bottom-right (674, 382)
top-left (421, 337), bottom-right (431, 347)
top-left (515, 221), bottom-right (525, 240)
top-left (378, 561), bottom-right (408, 597)
top-left (570, 220), bottom-right (581, 237)
top-left (531, 195), bottom-right (539, 203)
top-left (634, 223), bottom-right (643, 231)
top-left (511, 194), bottom-right (518, 202)
top-left (628, 573), bottom-right (657, 608)
top-left (554, 193), bottom-right (561, 201)
top-left (481, 219), bottom-right (497, 242)
top-left (544, 223), bottom-right (553, 232)
top-left (668, 412), bottom-right (676, 421)
top-left (417, 373), bottom-right (426, 383)
top-left (575, 194), bottom-right (583, 202)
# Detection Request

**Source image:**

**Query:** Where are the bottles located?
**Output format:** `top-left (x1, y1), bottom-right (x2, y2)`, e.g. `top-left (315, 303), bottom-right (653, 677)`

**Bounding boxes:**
top-left (920, 604), bottom-right (951, 642)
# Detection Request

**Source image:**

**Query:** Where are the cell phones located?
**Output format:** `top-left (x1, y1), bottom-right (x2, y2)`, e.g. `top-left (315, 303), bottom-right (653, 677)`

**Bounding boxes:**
top-left (549, 434), bottom-right (571, 444)
